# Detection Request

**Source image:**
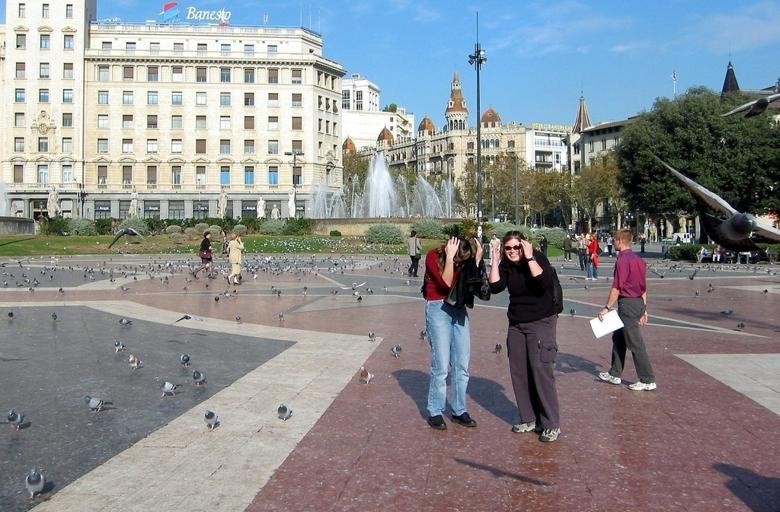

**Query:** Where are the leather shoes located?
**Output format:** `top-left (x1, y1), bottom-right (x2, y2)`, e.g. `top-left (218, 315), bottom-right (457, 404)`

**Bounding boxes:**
top-left (451, 412), bottom-right (476, 428)
top-left (427, 414), bottom-right (447, 430)
top-left (192, 271), bottom-right (241, 285)
top-left (413, 275), bottom-right (418, 277)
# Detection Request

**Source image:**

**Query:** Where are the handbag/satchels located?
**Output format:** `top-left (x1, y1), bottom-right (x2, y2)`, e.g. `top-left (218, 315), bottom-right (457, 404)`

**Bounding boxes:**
top-left (199, 250), bottom-right (211, 259)
top-left (414, 252), bottom-right (421, 259)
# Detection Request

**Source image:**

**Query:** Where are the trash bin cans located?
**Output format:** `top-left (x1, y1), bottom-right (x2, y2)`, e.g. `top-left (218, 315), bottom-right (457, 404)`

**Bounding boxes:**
top-left (662, 245), bottom-right (668, 257)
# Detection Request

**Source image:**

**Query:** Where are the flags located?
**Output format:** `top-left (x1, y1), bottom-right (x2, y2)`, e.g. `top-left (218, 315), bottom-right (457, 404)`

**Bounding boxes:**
top-left (163, 2), bottom-right (178, 22)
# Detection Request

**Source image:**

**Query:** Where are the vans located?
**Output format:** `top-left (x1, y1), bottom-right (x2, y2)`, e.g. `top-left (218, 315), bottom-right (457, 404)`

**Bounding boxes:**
top-left (672, 232), bottom-right (693, 244)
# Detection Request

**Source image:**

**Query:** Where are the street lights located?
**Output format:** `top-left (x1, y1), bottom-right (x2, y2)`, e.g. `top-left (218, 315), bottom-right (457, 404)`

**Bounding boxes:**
top-left (625, 213), bottom-right (634, 225)
top-left (39, 200), bottom-right (44, 217)
top-left (78, 191), bottom-right (88, 217)
top-left (466, 9), bottom-right (489, 242)
top-left (284, 150), bottom-right (306, 218)
top-left (507, 151), bottom-right (520, 224)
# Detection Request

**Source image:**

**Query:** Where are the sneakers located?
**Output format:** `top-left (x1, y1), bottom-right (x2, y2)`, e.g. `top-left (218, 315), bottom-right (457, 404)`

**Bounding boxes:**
top-left (598, 371), bottom-right (622, 385)
top-left (512, 420), bottom-right (536, 433)
top-left (627, 381), bottom-right (657, 392)
top-left (539, 427), bottom-right (562, 443)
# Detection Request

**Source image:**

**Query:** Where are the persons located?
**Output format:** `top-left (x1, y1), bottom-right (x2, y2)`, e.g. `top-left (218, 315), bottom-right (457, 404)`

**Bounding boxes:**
top-left (598, 229), bottom-right (657, 391)
top-left (47, 186), bottom-right (61, 219)
top-left (539, 234), bottom-right (547, 257)
top-left (192, 231), bottom-right (217, 280)
top-left (489, 233), bottom-right (500, 266)
top-left (696, 245), bottom-right (766, 265)
top-left (271, 203), bottom-right (281, 220)
top-left (218, 189), bottom-right (229, 217)
top-left (488, 230), bottom-right (561, 442)
top-left (563, 231), bottom-right (613, 281)
top-left (219, 230), bottom-right (229, 258)
top-left (129, 189), bottom-right (139, 218)
top-left (226, 233), bottom-right (245, 285)
top-left (407, 230), bottom-right (423, 277)
top-left (424, 223), bottom-right (484, 429)
top-left (289, 187), bottom-right (296, 218)
top-left (641, 236), bottom-right (646, 253)
top-left (257, 197), bottom-right (266, 219)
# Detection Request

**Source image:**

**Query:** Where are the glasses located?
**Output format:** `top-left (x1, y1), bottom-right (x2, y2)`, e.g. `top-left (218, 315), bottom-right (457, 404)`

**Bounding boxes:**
top-left (503, 244), bottom-right (521, 251)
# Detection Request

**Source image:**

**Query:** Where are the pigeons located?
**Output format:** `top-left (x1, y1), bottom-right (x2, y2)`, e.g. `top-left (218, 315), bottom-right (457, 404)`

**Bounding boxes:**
top-left (118, 318), bottom-right (135, 329)
top-left (570, 308), bottom-right (577, 318)
top-left (650, 150), bottom-right (780, 254)
top-left (368, 329), bottom-right (427, 360)
top-left (719, 93), bottom-right (780, 120)
top-left (81, 395), bottom-right (114, 417)
top-left (558, 264), bottom-right (611, 289)
top-left (277, 311), bottom-right (285, 321)
top-left (202, 409), bottom-right (219, 430)
top-left (495, 343), bottom-right (502, 354)
top-left (5, 409), bottom-right (26, 432)
top-left (47, 310), bottom-right (59, 323)
top-left (232, 313), bottom-right (242, 323)
top-left (720, 308), bottom-right (746, 329)
top-left (25, 463), bottom-right (48, 500)
top-left (278, 403), bottom-right (293, 421)
top-left (112, 339), bottom-right (208, 399)
top-left (358, 366), bottom-right (375, 384)
top-left (0, 227), bottom-right (413, 304)
top-left (173, 315), bottom-right (193, 325)
top-left (646, 258), bottom-right (780, 298)
top-left (6, 309), bottom-right (16, 321)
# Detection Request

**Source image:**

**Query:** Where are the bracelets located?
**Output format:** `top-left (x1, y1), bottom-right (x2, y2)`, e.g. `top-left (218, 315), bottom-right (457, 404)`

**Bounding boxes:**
top-left (526, 257), bottom-right (535, 262)
top-left (606, 305), bottom-right (609, 311)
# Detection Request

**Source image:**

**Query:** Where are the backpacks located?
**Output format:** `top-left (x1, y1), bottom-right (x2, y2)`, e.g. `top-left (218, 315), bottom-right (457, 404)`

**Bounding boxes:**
top-left (501, 251), bottom-right (563, 314)
